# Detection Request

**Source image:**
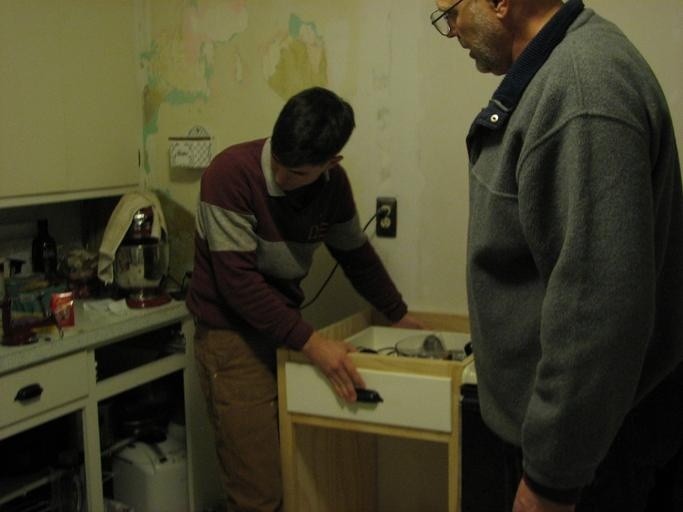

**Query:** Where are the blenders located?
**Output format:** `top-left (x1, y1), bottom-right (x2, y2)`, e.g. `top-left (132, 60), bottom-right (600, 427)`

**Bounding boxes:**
top-left (111, 207), bottom-right (167, 293)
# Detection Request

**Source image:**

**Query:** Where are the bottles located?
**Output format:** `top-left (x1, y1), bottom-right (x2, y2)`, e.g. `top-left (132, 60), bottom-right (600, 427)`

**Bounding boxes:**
top-left (31, 218), bottom-right (59, 275)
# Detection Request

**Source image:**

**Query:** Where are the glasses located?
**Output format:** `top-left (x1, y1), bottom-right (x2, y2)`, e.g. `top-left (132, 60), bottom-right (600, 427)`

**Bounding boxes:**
top-left (428, 0), bottom-right (464, 37)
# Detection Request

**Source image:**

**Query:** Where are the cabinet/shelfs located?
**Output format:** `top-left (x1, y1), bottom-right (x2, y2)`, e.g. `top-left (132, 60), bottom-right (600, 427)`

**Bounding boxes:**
top-left (276, 309), bottom-right (514, 511)
top-left (0, 177), bottom-right (205, 512)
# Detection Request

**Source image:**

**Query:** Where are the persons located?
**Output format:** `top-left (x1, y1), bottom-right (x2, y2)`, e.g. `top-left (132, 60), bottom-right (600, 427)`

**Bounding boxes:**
top-left (429, 1), bottom-right (682, 511)
top-left (182, 85), bottom-right (428, 511)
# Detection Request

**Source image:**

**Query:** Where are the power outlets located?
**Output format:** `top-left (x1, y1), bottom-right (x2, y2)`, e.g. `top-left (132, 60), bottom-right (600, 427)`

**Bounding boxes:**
top-left (375, 194), bottom-right (396, 241)
